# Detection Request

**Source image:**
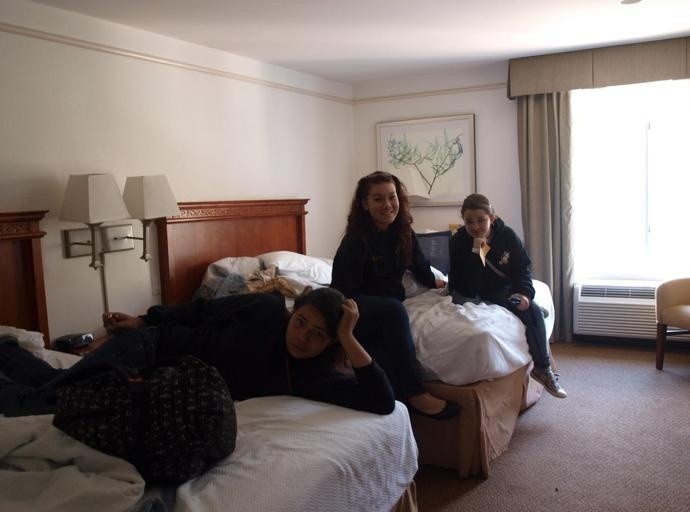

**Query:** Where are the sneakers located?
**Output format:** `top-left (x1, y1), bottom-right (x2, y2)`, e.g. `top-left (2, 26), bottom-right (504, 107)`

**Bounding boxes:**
top-left (531, 366), bottom-right (567, 398)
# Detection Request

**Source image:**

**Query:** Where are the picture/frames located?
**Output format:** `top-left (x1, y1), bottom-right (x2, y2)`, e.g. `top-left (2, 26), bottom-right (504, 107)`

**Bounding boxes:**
top-left (376, 113), bottom-right (476, 206)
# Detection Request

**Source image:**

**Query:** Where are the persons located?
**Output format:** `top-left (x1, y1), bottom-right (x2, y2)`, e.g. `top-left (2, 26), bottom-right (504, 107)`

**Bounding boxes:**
top-left (449, 194), bottom-right (568, 399)
top-left (0, 289), bottom-right (395, 416)
top-left (331, 172), bottom-right (461, 421)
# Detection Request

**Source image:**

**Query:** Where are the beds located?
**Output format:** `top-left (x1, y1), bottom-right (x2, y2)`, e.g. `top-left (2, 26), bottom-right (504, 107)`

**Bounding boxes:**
top-left (157, 199), bottom-right (555, 476)
top-left (0, 209), bottom-right (420, 512)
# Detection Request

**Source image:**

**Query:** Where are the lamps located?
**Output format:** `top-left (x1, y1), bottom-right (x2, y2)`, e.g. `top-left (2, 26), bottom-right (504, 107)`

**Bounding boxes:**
top-left (56, 172), bottom-right (130, 271)
top-left (122, 175), bottom-right (180, 263)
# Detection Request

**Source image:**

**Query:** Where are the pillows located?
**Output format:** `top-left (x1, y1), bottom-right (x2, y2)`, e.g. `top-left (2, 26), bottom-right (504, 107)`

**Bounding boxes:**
top-left (259, 251), bottom-right (333, 285)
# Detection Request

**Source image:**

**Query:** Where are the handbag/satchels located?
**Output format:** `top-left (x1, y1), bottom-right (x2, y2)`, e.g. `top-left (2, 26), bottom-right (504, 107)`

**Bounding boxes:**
top-left (53, 354), bottom-right (237, 483)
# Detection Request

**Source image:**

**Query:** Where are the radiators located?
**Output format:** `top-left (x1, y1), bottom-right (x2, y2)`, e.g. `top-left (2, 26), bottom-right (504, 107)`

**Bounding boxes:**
top-left (571, 281), bottom-right (690, 344)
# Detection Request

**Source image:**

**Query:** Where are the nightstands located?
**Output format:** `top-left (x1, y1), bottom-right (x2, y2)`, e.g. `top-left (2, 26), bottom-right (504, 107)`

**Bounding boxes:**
top-left (72, 334), bottom-right (109, 355)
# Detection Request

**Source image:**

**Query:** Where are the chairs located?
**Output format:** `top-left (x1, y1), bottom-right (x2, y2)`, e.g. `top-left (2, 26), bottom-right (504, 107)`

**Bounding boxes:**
top-left (655, 277), bottom-right (690, 370)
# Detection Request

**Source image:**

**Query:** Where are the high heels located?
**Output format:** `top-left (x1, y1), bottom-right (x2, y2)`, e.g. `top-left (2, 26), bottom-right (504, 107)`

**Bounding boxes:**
top-left (407, 400), bottom-right (461, 419)
